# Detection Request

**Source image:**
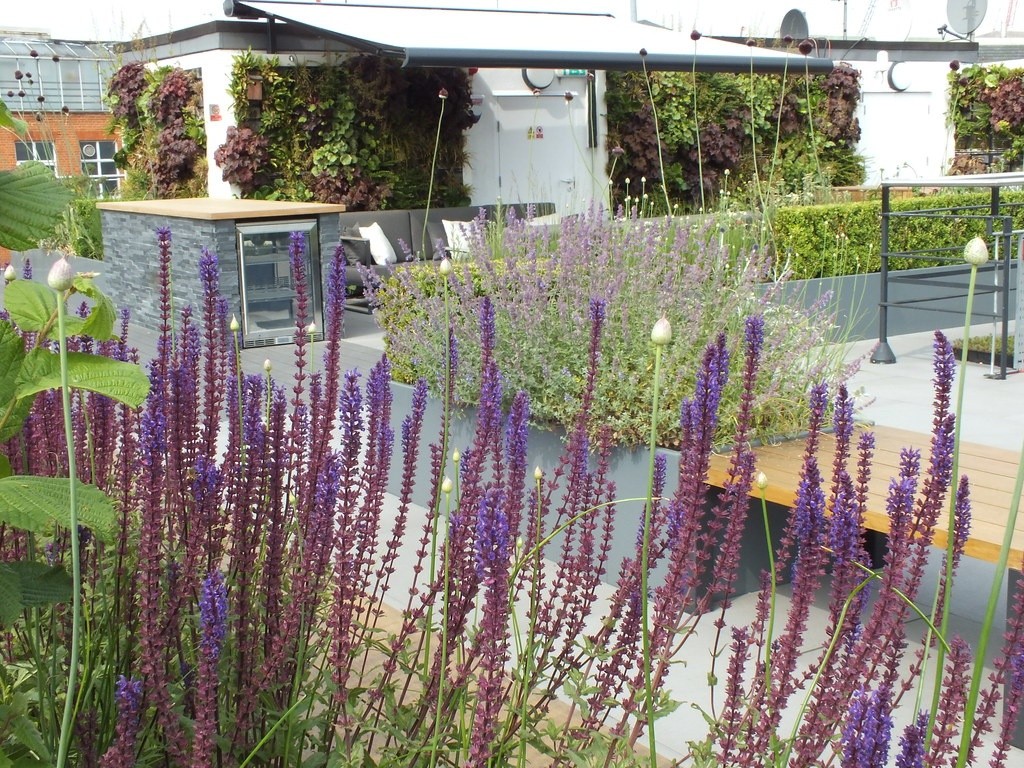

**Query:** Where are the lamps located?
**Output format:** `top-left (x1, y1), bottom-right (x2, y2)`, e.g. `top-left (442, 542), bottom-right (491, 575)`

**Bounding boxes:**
top-left (83, 145), bottom-right (95, 156)
top-left (887, 60), bottom-right (912, 91)
top-left (522, 68), bottom-right (553, 90)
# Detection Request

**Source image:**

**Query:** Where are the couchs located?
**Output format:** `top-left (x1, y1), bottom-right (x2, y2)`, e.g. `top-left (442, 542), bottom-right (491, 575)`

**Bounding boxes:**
top-left (338, 202), bottom-right (579, 318)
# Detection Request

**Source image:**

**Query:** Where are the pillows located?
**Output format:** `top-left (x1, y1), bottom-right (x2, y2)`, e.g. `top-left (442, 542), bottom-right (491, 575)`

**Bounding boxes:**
top-left (426, 221), bottom-right (452, 261)
top-left (441, 219), bottom-right (491, 261)
top-left (359, 222), bottom-right (397, 267)
top-left (532, 213), bottom-right (579, 240)
top-left (345, 223), bottom-right (361, 238)
